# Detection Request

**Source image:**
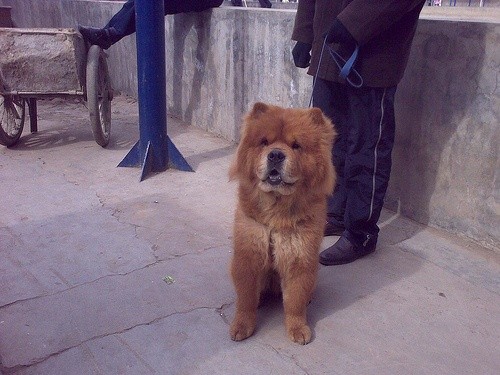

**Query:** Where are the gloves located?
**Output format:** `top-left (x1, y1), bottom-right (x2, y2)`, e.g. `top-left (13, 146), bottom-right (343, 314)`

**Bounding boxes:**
top-left (320, 18), bottom-right (355, 45)
top-left (290, 40), bottom-right (313, 69)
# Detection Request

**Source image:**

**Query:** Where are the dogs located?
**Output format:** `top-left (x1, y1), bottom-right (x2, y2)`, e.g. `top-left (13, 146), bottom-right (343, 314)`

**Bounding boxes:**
top-left (227, 101), bottom-right (338, 346)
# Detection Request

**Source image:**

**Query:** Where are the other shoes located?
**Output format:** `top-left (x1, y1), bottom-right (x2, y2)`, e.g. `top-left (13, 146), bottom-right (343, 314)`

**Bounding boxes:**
top-left (319, 221), bottom-right (379, 266)
top-left (77, 23), bottom-right (113, 50)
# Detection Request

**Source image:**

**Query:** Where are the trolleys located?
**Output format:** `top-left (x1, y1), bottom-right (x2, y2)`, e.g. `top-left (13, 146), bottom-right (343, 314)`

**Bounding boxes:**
top-left (0, 27), bottom-right (114, 147)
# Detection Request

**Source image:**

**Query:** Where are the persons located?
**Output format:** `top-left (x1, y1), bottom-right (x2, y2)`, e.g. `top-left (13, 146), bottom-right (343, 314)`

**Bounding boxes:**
top-left (77, 0), bottom-right (224, 51)
top-left (292, 0), bottom-right (426, 266)
